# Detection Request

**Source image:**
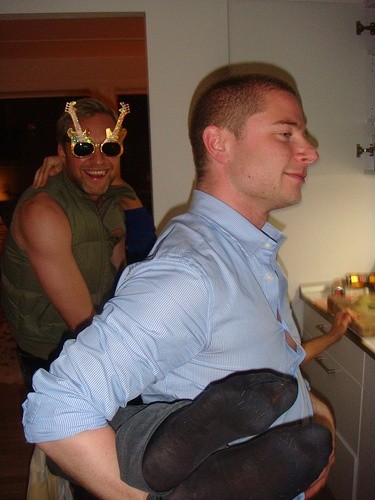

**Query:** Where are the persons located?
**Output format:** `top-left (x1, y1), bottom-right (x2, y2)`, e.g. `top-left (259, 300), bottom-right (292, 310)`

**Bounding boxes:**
top-left (17, 72), bottom-right (341, 500)
top-left (1, 97), bottom-right (138, 404)
top-left (31, 154), bottom-right (360, 500)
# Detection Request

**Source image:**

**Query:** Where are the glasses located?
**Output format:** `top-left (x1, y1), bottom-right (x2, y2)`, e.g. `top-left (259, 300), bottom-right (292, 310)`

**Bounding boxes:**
top-left (64, 101), bottom-right (131, 157)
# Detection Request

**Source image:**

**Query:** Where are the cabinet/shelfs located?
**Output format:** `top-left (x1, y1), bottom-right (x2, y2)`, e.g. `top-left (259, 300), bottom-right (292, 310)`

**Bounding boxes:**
top-left (300, 302), bottom-right (365, 500)
